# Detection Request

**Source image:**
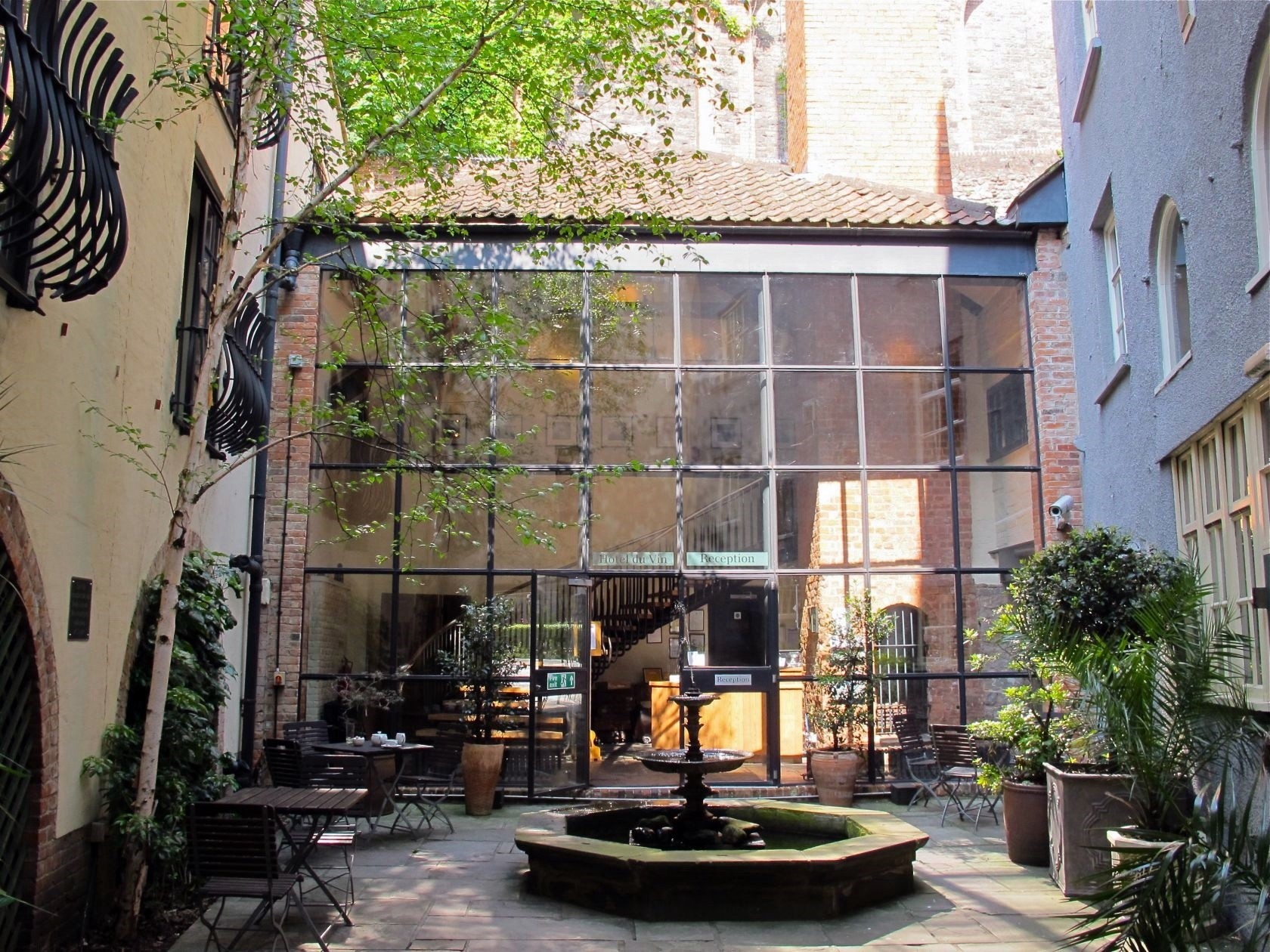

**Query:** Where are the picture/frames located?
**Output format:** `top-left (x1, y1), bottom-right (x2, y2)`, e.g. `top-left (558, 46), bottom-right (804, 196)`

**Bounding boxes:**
top-left (802, 398), bottom-right (816, 423)
top-left (775, 417), bottom-right (796, 449)
top-left (601, 415), bottom-right (634, 448)
top-left (656, 415), bottom-right (688, 449)
top-left (434, 413), bottom-right (469, 446)
top-left (496, 414), bottom-right (523, 441)
top-left (545, 413), bottom-right (579, 447)
top-left (710, 416), bottom-right (742, 449)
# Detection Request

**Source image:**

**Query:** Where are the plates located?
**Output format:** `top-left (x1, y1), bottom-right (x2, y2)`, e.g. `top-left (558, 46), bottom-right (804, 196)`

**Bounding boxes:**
top-left (381, 744), bottom-right (402, 748)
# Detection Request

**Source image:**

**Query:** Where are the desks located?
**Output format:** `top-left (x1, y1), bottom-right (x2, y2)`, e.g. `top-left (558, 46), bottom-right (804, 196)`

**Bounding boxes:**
top-left (214, 788), bottom-right (369, 952)
top-left (311, 741), bottom-right (434, 850)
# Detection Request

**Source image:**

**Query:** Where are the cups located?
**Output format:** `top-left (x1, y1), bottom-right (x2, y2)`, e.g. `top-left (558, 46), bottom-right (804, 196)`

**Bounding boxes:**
top-left (384, 739), bottom-right (398, 746)
top-left (396, 733), bottom-right (406, 746)
top-left (353, 736), bottom-right (365, 746)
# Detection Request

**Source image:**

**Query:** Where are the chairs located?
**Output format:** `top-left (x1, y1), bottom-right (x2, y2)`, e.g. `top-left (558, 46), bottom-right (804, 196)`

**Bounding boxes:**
top-left (282, 720), bottom-right (374, 832)
top-left (929, 722), bottom-right (999, 831)
top-left (183, 802), bottom-right (303, 952)
top-left (262, 736), bottom-right (349, 828)
top-left (892, 713), bottom-right (954, 815)
top-left (282, 754), bottom-right (369, 924)
top-left (389, 731), bottom-right (465, 841)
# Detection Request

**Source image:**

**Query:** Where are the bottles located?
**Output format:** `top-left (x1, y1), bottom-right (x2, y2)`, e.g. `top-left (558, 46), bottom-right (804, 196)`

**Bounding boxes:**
top-left (346, 737), bottom-right (353, 744)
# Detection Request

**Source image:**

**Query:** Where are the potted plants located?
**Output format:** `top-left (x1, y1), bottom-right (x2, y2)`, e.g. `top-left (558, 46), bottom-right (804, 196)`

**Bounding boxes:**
top-left (435, 584), bottom-right (525, 816)
top-left (808, 588), bottom-right (896, 806)
top-left (962, 525), bottom-right (1270, 952)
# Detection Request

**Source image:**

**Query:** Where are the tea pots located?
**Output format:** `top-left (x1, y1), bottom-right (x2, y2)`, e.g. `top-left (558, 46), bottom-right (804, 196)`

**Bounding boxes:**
top-left (371, 731), bottom-right (388, 747)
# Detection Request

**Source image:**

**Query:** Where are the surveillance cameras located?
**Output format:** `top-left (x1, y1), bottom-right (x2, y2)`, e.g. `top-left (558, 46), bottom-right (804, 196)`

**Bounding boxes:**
top-left (1048, 495), bottom-right (1076, 532)
